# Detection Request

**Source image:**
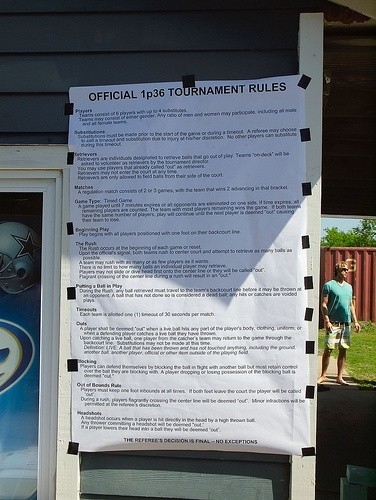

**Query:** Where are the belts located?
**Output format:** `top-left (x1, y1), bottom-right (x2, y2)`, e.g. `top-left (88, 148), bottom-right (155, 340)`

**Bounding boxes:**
top-left (331, 322), bottom-right (351, 326)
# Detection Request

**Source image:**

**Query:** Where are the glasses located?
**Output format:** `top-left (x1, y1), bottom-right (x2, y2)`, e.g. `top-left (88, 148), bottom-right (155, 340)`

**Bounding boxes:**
top-left (339, 268), bottom-right (348, 271)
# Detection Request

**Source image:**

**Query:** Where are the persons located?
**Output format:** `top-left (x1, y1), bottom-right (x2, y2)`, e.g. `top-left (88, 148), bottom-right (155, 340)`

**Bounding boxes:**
top-left (318, 261), bottom-right (361, 386)
